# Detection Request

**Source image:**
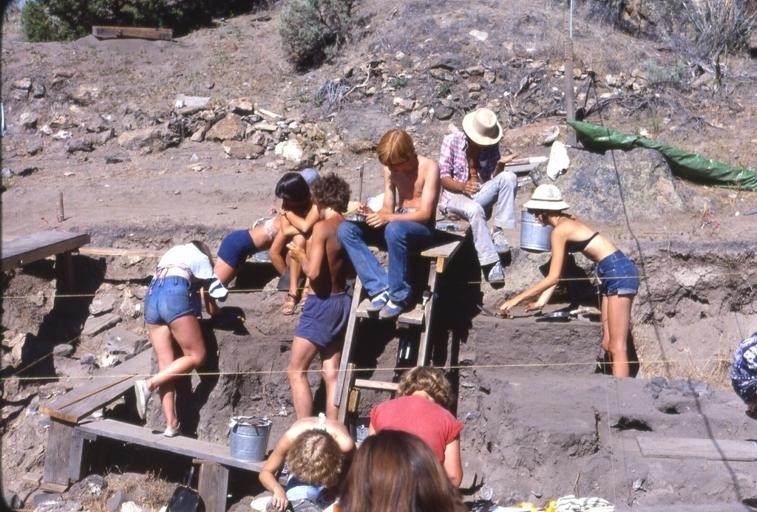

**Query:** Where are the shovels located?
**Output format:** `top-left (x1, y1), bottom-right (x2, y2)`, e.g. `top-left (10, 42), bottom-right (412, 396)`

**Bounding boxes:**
top-left (544, 308), bottom-right (590, 319)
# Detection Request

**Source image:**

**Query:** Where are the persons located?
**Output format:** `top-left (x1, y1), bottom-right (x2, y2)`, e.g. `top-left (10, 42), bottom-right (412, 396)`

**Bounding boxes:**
top-left (731, 329), bottom-right (757, 420)
top-left (285, 171), bottom-right (352, 421)
top-left (438, 107), bottom-right (519, 285)
top-left (257, 416), bottom-right (355, 510)
top-left (133, 239), bottom-right (227, 437)
top-left (318, 428), bottom-right (471, 512)
top-left (367, 365), bottom-right (464, 490)
top-left (214, 128), bottom-right (638, 377)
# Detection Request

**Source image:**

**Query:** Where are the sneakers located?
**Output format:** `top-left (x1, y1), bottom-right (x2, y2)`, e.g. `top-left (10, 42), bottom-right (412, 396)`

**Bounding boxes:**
top-left (487, 262), bottom-right (504, 284)
top-left (135, 380), bottom-right (152, 420)
top-left (164, 422), bottom-right (183, 437)
top-left (490, 229), bottom-right (509, 254)
top-left (379, 300), bottom-right (407, 318)
top-left (367, 289), bottom-right (388, 311)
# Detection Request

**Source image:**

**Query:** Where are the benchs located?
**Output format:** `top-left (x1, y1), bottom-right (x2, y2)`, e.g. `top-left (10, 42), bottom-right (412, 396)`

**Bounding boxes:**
top-left (329, 209), bottom-right (472, 410)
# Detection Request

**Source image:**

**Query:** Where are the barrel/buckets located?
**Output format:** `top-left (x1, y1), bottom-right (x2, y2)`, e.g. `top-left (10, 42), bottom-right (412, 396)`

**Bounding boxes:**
top-left (519, 210), bottom-right (553, 251)
top-left (226, 416), bottom-right (273, 461)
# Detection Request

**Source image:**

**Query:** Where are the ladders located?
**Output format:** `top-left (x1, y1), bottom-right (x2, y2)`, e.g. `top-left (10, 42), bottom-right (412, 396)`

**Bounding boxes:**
top-left (334, 241), bottom-right (436, 427)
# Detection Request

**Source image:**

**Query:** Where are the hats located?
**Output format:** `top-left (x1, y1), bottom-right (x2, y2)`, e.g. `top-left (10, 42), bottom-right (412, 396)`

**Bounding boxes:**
top-left (523, 184), bottom-right (569, 210)
top-left (462, 108), bottom-right (503, 146)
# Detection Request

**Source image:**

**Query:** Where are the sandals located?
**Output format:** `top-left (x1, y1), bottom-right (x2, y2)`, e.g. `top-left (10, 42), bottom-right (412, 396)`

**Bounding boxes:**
top-left (295, 299), bottom-right (305, 315)
top-left (282, 293), bottom-right (299, 315)
